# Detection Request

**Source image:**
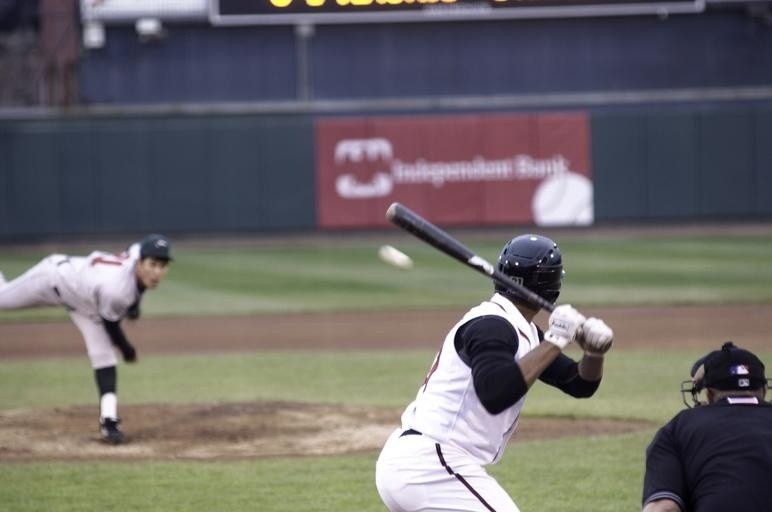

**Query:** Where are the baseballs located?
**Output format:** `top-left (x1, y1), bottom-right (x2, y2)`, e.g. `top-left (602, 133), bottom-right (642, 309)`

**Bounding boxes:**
top-left (380, 245), bottom-right (412, 268)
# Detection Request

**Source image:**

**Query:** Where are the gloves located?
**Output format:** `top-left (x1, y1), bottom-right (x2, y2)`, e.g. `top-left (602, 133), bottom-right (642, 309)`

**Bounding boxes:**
top-left (580, 317), bottom-right (613, 358)
top-left (543, 304), bottom-right (585, 351)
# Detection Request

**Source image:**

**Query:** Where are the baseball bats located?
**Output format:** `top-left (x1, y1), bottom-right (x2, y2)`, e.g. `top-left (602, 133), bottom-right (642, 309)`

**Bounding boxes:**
top-left (386, 202), bottom-right (586, 336)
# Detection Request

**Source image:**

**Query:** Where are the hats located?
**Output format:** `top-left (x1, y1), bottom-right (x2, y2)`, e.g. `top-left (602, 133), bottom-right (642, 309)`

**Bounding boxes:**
top-left (141, 234), bottom-right (172, 262)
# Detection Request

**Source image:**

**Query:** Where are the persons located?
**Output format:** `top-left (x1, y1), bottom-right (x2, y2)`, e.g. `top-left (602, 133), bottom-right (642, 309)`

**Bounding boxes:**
top-left (639, 342), bottom-right (772, 512)
top-left (374, 234), bottom-right (612, 512)
top-left (1, 233), bottom-right (174, 442)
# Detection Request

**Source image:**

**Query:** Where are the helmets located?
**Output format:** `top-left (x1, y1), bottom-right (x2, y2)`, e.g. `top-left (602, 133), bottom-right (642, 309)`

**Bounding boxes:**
top-left (681, 342), bottom-right (771, 408)
top-left (494, 233), bottom-right (565, 311)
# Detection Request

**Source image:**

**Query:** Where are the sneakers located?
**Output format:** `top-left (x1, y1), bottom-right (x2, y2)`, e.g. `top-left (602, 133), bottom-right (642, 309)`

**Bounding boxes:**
top-left (99, 417), bottom-right (123, 443)
